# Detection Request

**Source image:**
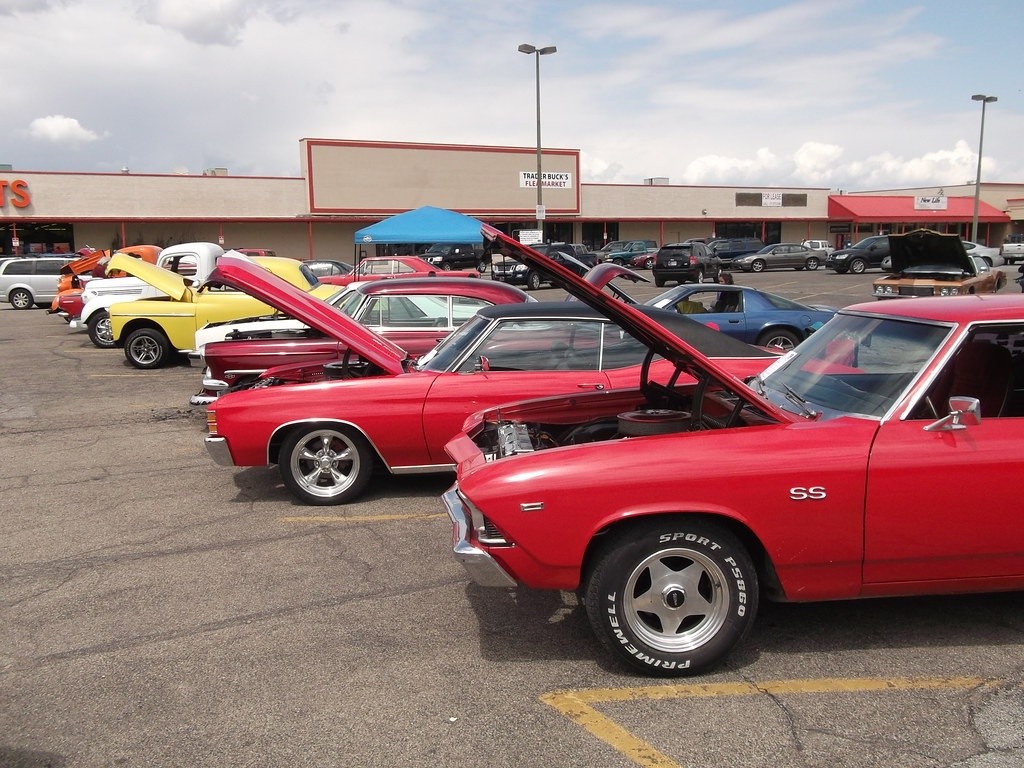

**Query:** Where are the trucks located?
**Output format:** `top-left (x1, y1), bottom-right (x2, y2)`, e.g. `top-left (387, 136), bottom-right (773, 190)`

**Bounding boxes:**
top-left (490, 244), bottom-right (598, 291)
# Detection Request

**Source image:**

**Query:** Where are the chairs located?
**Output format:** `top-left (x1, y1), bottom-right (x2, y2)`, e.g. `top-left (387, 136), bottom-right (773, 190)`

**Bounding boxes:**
top-left (431, 317), bottom-right (448, 327)
top-left (914, 345), bottom-right (955, 419)
top-left (538, 339), bottom-right (568, 370)
top-left (555, 348), bottom-right (586, 369)
top-left (722, 293), bottom-right (739, 312)
top-left (938, 341), bottom-right (1016, 418)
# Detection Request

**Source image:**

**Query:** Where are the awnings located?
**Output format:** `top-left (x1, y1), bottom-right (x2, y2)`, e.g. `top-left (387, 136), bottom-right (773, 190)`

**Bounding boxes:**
top-left (828, 195), bottom-right (1010, 223)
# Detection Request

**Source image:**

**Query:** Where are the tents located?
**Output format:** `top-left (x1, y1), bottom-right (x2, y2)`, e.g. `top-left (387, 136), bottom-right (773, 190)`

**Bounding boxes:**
top-left (353, 205), bottom-right (485, 282)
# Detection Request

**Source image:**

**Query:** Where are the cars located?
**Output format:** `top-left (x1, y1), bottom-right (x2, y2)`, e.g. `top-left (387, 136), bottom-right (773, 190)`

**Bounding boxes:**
top-left (202, 255), bottom-right (867, 506)
top-left (303, 258), bottom-right (355, 276)
top-left (827, 235), bottom-right (891, 274)
top-left (556, 250), bottom-right (844, 350)
top-left (105, 254), bottom-right (348, 370)
top-left (440, 222), bottom-right (1023, 679)
top-left (731, 243), bottom-right (828, 273)
top-left (419, 242), bottom-right (491, 273)
top-left (571, 236), bottom-right (764, 269)
top-left (880, 240), bottom-right (1005, 274)
top-left (1002, 233), bottom-right (1024, 265)
top-left (870, 227), bottom-right (1007, 300)
top-left (189, 262), bottom-right (651, 406)
top-left (0, 243), bottom-right (277, 349)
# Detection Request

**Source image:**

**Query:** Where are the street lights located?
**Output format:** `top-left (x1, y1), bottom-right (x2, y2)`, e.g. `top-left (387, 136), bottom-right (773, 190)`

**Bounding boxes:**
top-left (971, 94), bottom-right (997, 243)
top-left (518, 43), bottom-right (557, 229)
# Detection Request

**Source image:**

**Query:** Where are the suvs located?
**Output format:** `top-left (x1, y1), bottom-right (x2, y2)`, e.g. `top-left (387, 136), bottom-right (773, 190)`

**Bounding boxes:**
top-left (801, 238), bottom-right (836, 256)
top-left (652, 242), bottom-right (723, 288)
